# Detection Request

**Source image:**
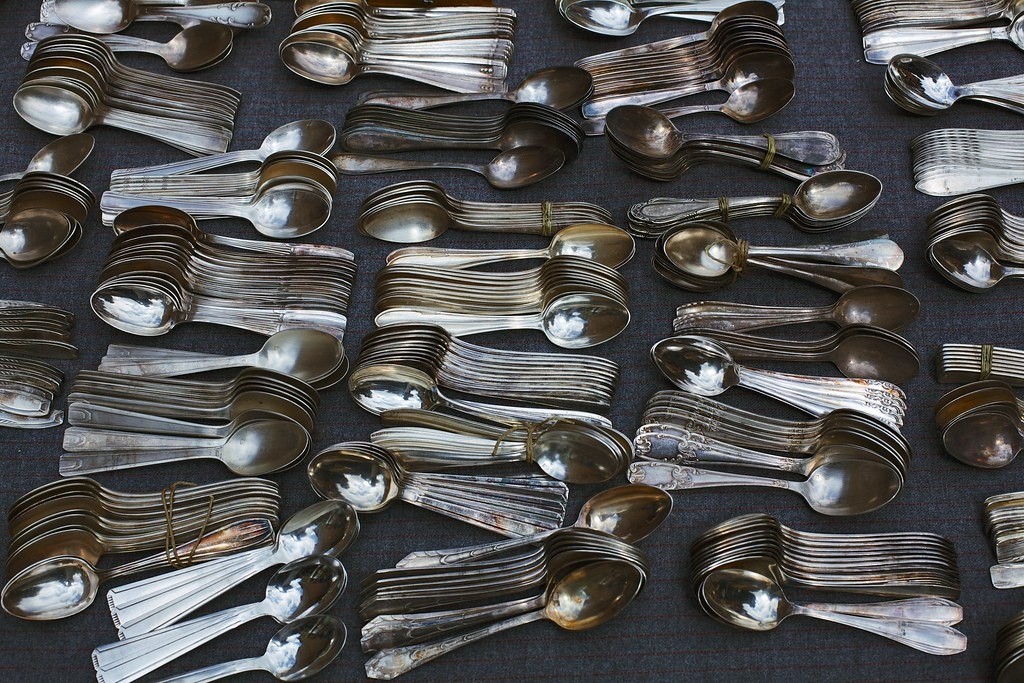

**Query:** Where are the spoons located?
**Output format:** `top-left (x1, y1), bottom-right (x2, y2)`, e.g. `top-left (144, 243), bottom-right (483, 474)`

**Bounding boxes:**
top-left (627, 284), bottom-right (921, 516)
top-left (627, 170), bottom-right (904, 294)
top-left (100, 119), bottom-right (338, 239)
top-left (936, 380), bottom-right (1024, 469)
top-left (332, 65), bottom-right (595, 190)
top-left (359, 484), bottom-right (674, 679)
top-left (355, 180), bottom-right (615, 243)
top-left (308, 441), bottom-right (569, 538)
top-left (603, 105), bottom-right (848, 181)
top-left (90, 204), bottom-right (355, 342)
top-left (373, 223), bottom-right (636, 349)
top-left (348, 321), bottom-right (619, 428)
top-left (996, 610), bottom-right (1024, 683)
top-left (20, 0), bottom-right (272, 72)
top-left (371, 408), bottom-right (636, 484)
top-left (1, 477), bottom-right (361, 641)
top-left (13, 34), bottom-right (241, 158)
top-left (554, 0), bottom-right (796, 136)
top-left (924, 194), bottom-right (1024, 293)
top-left (59, 328), bottom-right (350, 477)
top-left (851, 0), bottom-right (1024, 114)
top-left (690, 513), bottom-right (967, 656)
top-left (0, 134), bottom-right (96, 269)
top-left (92, 554), bottom-right (348, 683)
top-left (279, 0), bottom-right (517, 94)
